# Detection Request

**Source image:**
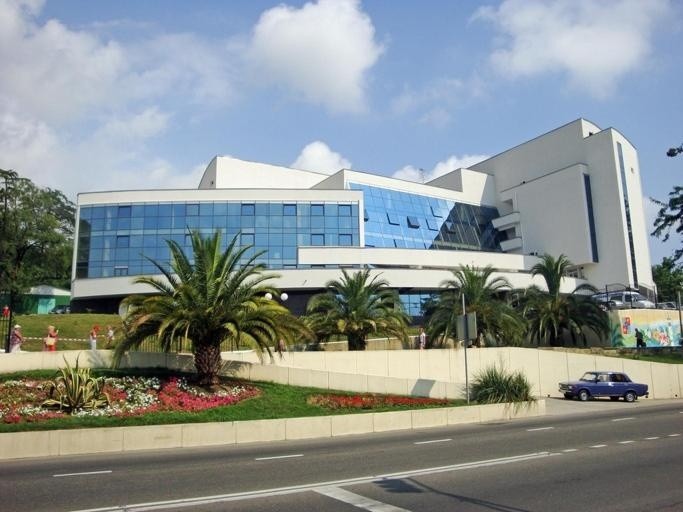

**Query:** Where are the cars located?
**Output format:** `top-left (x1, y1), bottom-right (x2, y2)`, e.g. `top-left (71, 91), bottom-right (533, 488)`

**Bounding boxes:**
top-left (556, 370), bottom-right (649, 403)
top-left (48, 304), bottom-right (72, 314)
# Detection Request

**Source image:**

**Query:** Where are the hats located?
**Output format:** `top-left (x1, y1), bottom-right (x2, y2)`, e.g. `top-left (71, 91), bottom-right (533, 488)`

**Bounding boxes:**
top-left (13, 324), bottom-right (21, 329)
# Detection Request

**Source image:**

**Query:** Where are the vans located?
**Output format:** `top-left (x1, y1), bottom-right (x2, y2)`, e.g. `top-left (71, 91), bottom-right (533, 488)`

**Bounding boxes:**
top-left (590, 290), bottom-right (683, 310)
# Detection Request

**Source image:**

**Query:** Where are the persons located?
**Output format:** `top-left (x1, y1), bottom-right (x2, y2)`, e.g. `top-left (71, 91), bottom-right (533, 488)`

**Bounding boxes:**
top-left (103, 325), bottom-right (115, 344)
top-left (45, 325), bottom-right (58, 351)
top-left (1, 303), bottom-right (9, 320)
top-left (634, 327), bottom-right (644, 348)
top-left (87, 324), bottom-right (100, 349)
top-left (43, 334), bottom-right (49, 351)
top-left (7, 323), bottom-right (24, 352)
top-left (417, 327), bottom-right (426, 349)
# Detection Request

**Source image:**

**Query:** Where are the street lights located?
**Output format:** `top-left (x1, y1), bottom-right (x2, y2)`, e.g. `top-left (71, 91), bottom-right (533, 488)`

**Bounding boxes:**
top-left (264, 292), bottom-right (289, 304)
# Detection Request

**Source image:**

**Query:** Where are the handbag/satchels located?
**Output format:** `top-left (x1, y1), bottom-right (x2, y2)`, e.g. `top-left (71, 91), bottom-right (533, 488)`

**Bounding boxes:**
top-left (45, 337), bottom-right (55, 346)
